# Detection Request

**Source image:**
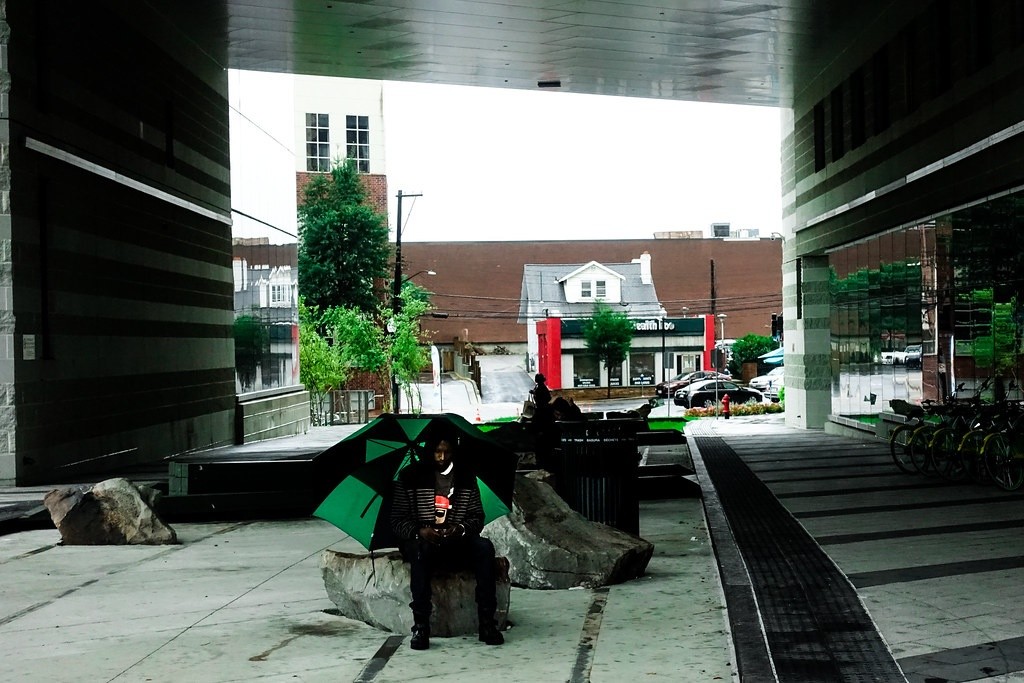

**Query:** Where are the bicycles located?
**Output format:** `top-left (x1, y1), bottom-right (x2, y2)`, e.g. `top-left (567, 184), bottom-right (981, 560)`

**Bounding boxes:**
top-left (883, 356), bottom-right (1024, 492)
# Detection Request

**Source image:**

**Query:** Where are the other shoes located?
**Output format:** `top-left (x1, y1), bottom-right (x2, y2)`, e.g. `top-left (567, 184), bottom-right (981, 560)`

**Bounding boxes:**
top-left (410, 622), bottom-right (431, 649)
top-left (479, 619), bottom-right (504, 645)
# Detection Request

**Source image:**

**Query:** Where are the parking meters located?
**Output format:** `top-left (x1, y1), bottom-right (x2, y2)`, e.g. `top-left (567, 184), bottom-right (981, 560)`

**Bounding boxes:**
top-left (640, 375), bottom-right (645, 397)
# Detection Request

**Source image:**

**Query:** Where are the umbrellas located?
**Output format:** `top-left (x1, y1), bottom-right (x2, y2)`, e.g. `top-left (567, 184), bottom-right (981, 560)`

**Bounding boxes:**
top-left (306, 413), bottom-right (514, 562)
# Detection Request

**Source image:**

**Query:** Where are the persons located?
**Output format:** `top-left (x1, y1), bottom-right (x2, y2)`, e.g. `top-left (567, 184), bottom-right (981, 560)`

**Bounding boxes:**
top-left (536, 395), bottom-right (584, 432)
top-left (389, 434), bottom-right (505, 651)
top-left (528, 373), bottom-right (554, 414)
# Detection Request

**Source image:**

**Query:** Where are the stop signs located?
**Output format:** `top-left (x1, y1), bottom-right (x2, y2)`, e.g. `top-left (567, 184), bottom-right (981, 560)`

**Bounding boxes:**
top-left (881, 330), bottom-right (889, 339)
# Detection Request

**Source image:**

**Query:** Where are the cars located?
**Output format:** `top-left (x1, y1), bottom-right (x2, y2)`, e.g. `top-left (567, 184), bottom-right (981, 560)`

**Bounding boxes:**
top-left (748, 366), bottom-right (784, 391)
top-left (673, 377), bottom-right (762, 408)
top-left (655, 368), bottom-right (731, 399)
top-left (764, 372), bottom-right (785, 402)
top-left (903, 345), bottom-right (923, 364)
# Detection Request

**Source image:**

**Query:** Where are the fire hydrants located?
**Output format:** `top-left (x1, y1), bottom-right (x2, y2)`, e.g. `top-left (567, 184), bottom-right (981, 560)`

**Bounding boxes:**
top-left (722, 394), bottom-right (731, 419)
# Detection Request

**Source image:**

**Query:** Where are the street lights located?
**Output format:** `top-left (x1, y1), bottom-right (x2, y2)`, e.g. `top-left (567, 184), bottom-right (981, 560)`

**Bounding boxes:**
top-left (393, 270), bottom-right (448, 413)
top-left (717, 314), bottom-right (726, 372)
